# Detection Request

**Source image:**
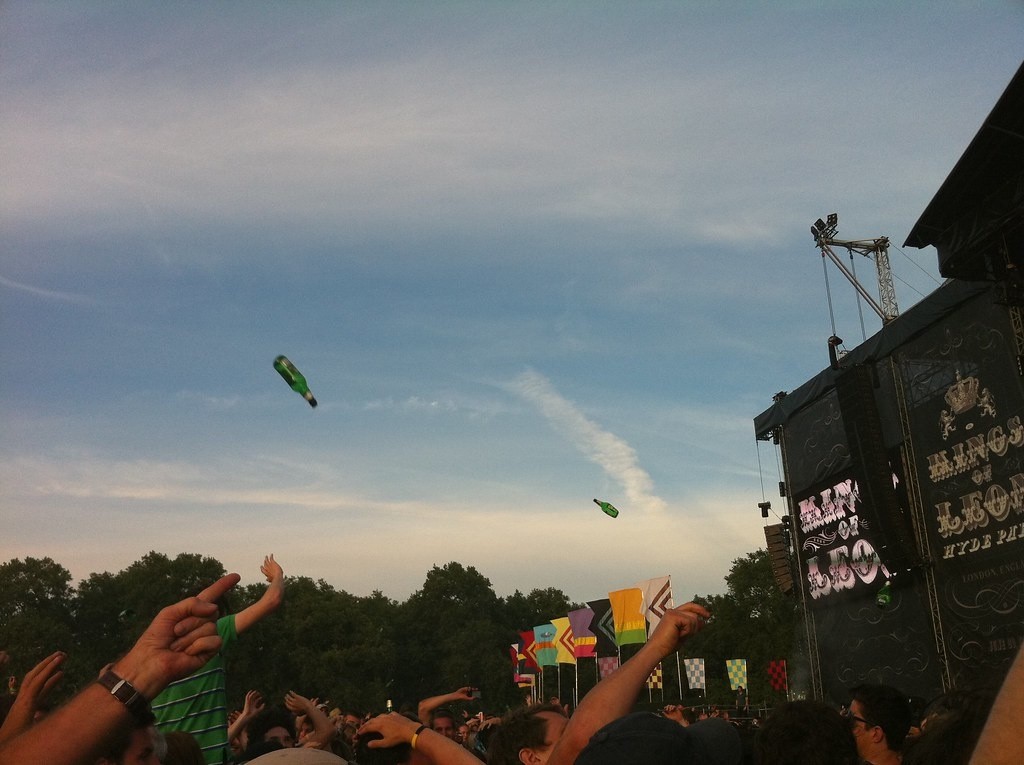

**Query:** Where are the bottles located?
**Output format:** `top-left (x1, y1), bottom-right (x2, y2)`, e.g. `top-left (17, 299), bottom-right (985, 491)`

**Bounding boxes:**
top-left (273, 355), bottom-right (318, 408)
top-left (592, 498), bottom-right (619, 519)
top-left (466, 682), bottom-right (473, 697)
top-left (876, 584), bottom-right (894, 609)
top-left (386, 696), bottom-right (393, 714)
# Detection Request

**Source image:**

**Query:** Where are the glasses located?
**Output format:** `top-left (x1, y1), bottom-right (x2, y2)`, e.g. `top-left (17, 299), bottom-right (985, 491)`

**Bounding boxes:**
top-left (844, 710), bottom-right (875, 729)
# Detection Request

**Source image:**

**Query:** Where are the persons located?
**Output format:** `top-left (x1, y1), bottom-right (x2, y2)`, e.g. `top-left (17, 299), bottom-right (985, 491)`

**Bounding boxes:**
top-left (0, 554), bottom-right (1024, 765)
top-left (734, 686), bottom-right (747, 717)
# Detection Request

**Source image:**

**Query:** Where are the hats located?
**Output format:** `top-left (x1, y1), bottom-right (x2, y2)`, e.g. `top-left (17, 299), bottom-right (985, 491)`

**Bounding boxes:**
top-left (316, 704), bottom-right (328, 710)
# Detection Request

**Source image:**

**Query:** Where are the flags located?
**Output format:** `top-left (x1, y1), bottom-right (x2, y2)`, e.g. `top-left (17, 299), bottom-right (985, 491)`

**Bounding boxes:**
top-left (534, 624), bottom-right (560, 666)
top-left (647, 662), bottom-right (663, 688)
top-left (609, 588), bottom-right (647, 645)
top-left (598, 657), bottom-right (618, 679)
top-left (509, 643), bottom-right (532, 684)
top-left (726, 659), bottom-right (748, 691)
top-left (567, 608), bottom-right (597, 657)
top-left (684, 658), bottom-right (705, 689)
top-left (517, 630), bottom-right (540, 673)
top-left (518, 674), bottom-right (536, 688)
top-left (768, 660), bottom-right (788, 691)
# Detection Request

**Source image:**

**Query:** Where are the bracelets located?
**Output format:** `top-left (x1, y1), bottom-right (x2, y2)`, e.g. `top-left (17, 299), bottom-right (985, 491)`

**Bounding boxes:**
top-left (412, 725), bottom-right (428, 750)
top-left (98, 670), bottom-right (152, 722)
